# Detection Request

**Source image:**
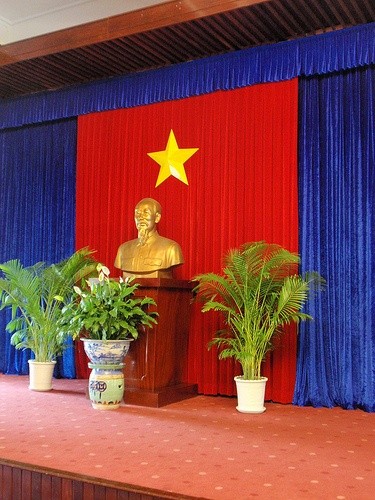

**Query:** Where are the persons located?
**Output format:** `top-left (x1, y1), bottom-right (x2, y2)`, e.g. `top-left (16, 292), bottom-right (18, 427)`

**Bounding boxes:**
top-left (114, 197), bottom-right (184, 272)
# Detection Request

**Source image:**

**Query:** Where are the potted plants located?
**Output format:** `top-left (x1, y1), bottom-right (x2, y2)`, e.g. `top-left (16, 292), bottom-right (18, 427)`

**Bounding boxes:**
top-left (53, 264), bottom-right (159, 411)
top-left (187, 241), bottom-right (327, 411)
top-left (1, 243), bottom-right (100, 392)
